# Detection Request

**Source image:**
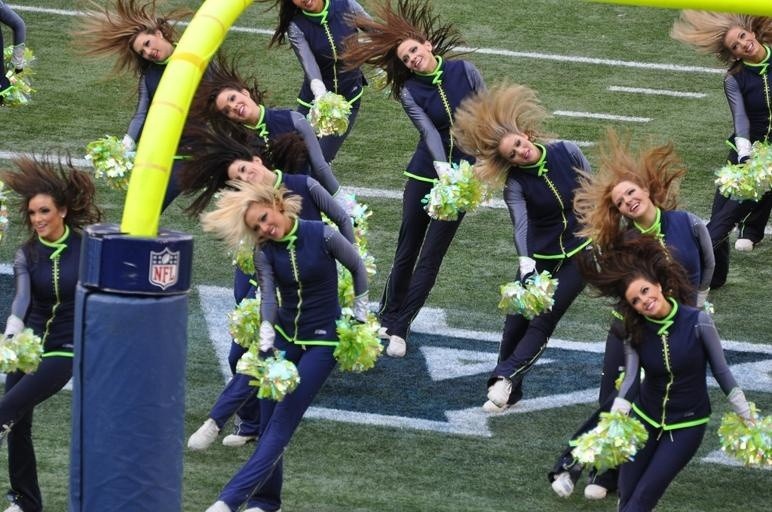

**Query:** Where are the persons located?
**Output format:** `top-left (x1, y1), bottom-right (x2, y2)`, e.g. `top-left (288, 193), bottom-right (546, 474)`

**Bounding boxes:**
top-left (548, 126), bottom-right (715, 499)
top-left (735, 201), bottom-right (772, 252)
top-left (196, 179), bottom-right (371, 512)
top-left (1, 0), bottom-right (24, 100)
top-left (67, 0), bottom-right (485, 512)
top-left (585, 233), bottom-right (758, 510)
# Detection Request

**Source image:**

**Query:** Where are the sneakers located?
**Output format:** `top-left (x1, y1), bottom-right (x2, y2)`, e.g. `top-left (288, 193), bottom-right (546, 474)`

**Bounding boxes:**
top-left (551, 471), bottom-right (574, 499)
top-left (188, 418), bottom-right (220, 450)
top-left (584, 484), bottom-right (608, 500)
top-left (386, 335), bottom-right (406, 358)
top-left (223, 433), bottom-right (259, 447)
top-left (482, 376), bottom-right (513, 412)
top-left (204, 500), bottom-right (232, 512)
top-left (734, 239), bottom-right (753, 252)
